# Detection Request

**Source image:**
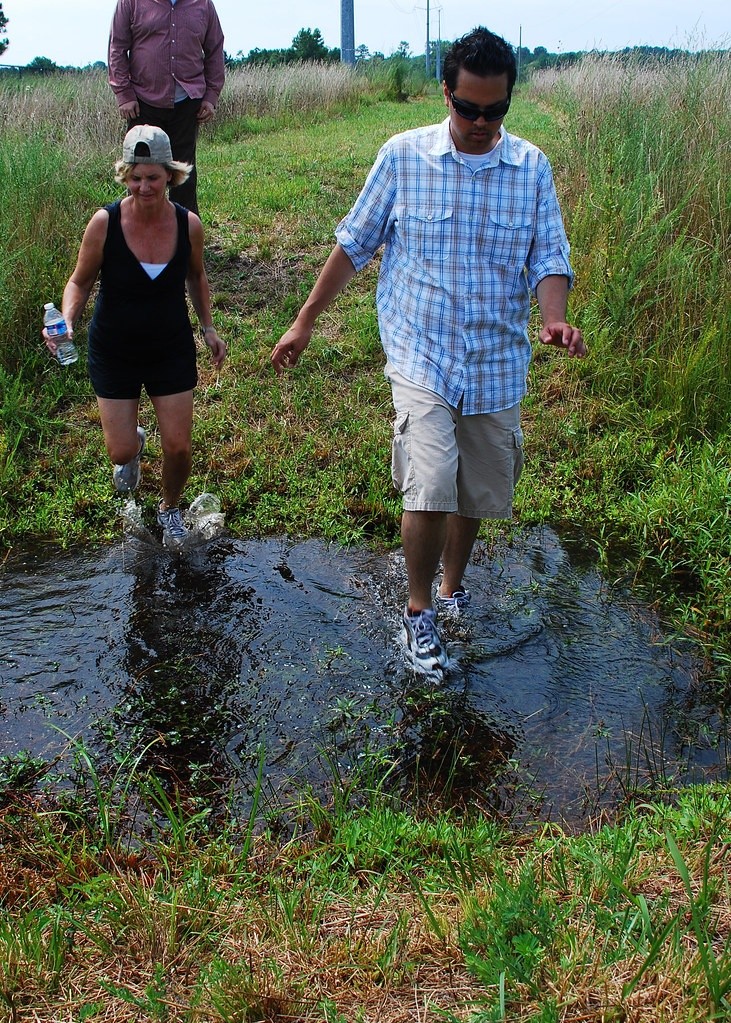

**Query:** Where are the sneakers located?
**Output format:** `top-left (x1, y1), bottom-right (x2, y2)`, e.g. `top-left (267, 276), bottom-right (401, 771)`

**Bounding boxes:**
top-left (111, 427), bottom-right (144, 491)
top-left (401, 609), bottom-right (449, 669)
top-left (156, 499), bottom-right (189, 547)
top-left (438, 583), bottom-right (474, 616)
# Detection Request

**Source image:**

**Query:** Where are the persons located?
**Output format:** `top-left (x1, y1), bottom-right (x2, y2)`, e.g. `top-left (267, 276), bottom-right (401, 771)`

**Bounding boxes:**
top-left (41, 123), bottom-right (226, 553)
top-left (106, 1), bottom-right (225, 219)
top-left (267, 26), bottom-right (590, 680)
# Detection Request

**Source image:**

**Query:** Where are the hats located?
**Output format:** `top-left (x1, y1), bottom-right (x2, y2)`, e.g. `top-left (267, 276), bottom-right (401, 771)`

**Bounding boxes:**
top-left (121, 125), bottom-right (171, 164)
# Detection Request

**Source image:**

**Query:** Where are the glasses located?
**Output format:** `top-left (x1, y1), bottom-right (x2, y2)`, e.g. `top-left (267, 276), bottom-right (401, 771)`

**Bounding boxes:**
top-left (448, 90), bottom-right (515, 122)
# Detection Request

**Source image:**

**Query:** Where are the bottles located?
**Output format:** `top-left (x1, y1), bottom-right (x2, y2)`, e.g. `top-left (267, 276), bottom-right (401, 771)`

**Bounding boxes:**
top-left (43, 302), bottom-right (79, 365)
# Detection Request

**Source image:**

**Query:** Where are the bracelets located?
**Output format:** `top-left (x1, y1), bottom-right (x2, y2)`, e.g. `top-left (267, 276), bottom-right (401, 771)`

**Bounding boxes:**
top-left (200, 327), bottom-right (216, 335)
